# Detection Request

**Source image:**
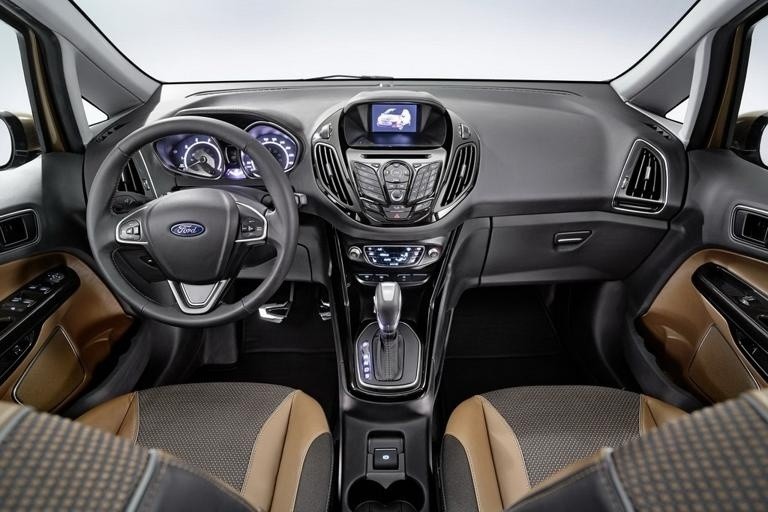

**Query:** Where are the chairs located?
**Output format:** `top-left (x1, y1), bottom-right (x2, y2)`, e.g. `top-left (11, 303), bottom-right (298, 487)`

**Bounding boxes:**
top-left (441, 384), bottom-right (767, 512)
top-left (0, 383), bottom-right (336, 512)
